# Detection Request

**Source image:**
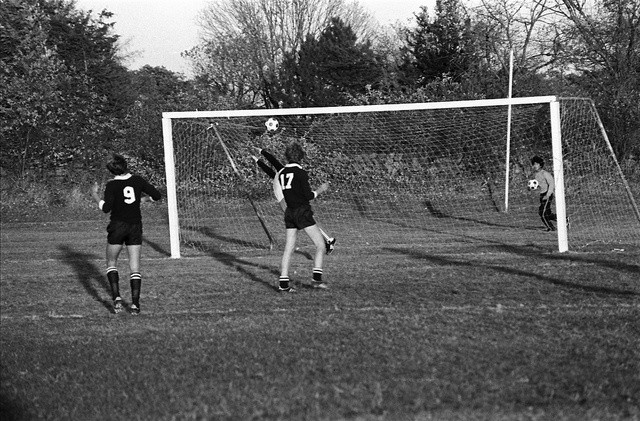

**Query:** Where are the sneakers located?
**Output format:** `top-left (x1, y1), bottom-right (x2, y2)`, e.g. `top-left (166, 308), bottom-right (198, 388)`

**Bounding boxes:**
top-left (324, 237), bottom-right (337, 255)
top-left (130, 304), bottom-right (140, 316)
top-left (310, 279), bottom-right (331, 290)
top-left (113, 297), bottom-right (123, 314)
top-left (277, 285), bottom-right (297, 294)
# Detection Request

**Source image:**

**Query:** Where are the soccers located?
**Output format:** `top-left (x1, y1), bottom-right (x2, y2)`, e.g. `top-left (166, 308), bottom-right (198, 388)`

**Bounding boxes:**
top-left (527, 179), bottom-right (539, 190)
top-left (265, 117), bottom-right (279, 132)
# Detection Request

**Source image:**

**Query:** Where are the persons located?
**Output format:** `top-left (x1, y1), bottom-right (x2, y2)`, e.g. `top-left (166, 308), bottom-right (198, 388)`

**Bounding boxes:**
top-left (250, 145), bottom-right (336, 255)
top-left (278, 143), bottom-right (331, 293)
top-left (527, 156), bottom-right (571, 233)
top-left (91, 153), bottom-right (161, 316)
